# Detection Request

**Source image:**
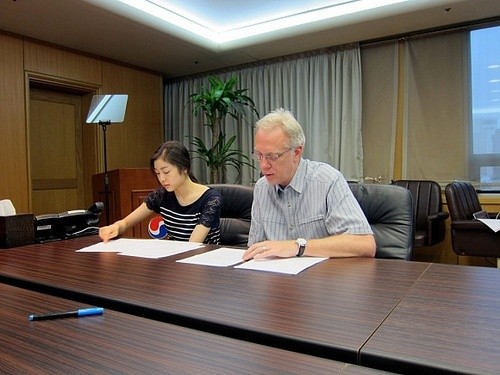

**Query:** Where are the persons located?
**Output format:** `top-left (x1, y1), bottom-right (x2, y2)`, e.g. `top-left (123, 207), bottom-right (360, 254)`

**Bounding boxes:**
top-left (242, 108), bottom-right (377, 260)
top-left (99, 141), bottom-right (221, 245)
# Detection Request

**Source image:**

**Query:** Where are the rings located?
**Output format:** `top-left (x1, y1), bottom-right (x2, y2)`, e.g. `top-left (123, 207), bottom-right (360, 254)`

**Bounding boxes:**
top-left (263, 246), bottom-right (266, 251)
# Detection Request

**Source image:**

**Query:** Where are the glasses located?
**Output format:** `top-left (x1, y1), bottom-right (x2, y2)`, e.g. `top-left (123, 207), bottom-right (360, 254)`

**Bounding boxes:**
top-left (250, 146), bottom-right (295, 162)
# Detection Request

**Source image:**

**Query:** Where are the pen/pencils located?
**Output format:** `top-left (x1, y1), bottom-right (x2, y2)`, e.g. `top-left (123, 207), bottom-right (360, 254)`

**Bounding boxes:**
top-left (29, 307), bottom-right (104, 321)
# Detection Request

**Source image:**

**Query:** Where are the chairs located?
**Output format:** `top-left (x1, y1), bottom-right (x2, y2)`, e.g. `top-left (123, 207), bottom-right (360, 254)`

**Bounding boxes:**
top-left (348, 181), bottom-right (413, 261)
top-left (443, 180), bottom-right (500, 269)
top-left (203, 182), bottom-right (254, 247)
top-left (389, 179), bottom-right (449, 247)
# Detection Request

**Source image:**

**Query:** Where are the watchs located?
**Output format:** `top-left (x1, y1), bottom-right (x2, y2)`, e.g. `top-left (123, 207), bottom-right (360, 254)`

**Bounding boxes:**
top-left (295, 237), bottom-right (307, 257)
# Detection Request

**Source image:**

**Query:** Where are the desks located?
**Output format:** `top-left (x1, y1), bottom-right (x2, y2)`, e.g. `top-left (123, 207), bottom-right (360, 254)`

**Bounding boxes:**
top-left (0, 234), bottom-right (500, 375)
